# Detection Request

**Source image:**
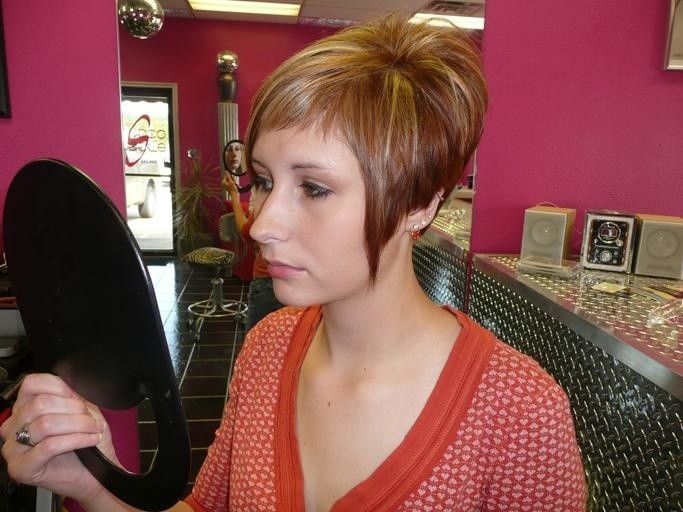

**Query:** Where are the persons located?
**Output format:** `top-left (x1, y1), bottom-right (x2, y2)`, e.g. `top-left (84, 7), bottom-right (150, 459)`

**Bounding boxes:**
top-left (220, 170), bottom-right (288, 333)
top-left (0, 13), bottom-right (588, 512)
top-left (225, 142), bottom-right (241, 173)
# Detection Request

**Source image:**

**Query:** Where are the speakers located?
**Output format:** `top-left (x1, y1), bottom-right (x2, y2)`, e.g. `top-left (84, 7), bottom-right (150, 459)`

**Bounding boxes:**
top-left (519, 205), bottom-right (576, 269)
top-left (633, 212), bottom-right (683, 280)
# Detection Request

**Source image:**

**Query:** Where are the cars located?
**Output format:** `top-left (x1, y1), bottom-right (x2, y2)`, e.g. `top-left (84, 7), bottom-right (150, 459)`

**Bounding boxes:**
top-left (121, 128), bottom-right (162, 219)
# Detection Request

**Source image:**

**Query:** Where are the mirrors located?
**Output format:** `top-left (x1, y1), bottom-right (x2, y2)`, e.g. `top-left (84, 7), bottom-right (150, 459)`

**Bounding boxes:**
top-left (114, 1), bottom-right (486, 488)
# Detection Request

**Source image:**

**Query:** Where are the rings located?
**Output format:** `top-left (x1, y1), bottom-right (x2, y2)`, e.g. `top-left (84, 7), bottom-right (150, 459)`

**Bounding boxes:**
top-left (14, 423), bottom-right (36, 447)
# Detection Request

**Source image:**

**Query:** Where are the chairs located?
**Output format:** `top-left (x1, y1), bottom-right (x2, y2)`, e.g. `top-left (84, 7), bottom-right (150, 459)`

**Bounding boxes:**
top-left (181, 212), bottom-right (248, 318)
